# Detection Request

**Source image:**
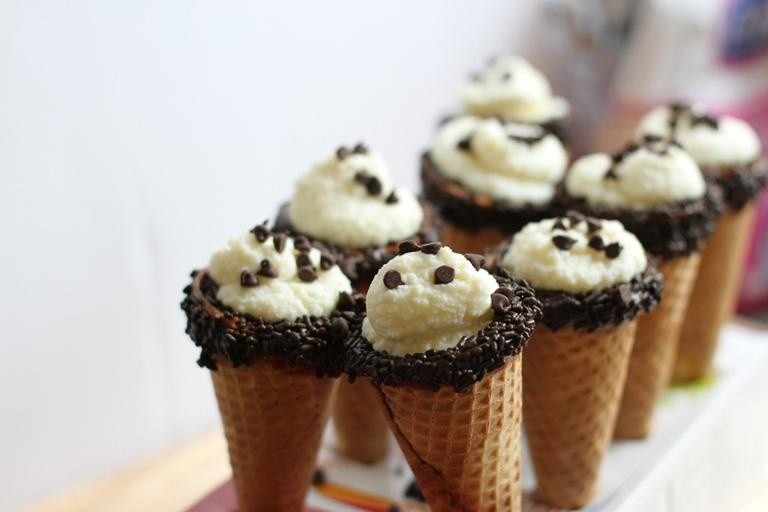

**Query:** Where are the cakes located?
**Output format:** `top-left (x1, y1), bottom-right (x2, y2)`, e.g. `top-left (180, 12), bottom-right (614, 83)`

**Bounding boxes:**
top-left (183, 52), bottom-right (767, 511)
